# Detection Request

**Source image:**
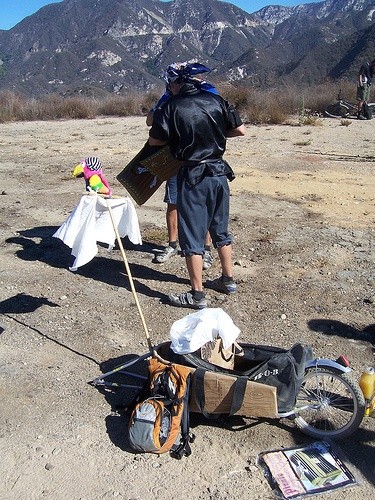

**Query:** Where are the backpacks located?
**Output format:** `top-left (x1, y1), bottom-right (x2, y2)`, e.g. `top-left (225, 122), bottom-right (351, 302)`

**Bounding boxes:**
top-left (128, 359), bottom-right (196, 459)
top-left (363, 102), bottom-right (372, 119)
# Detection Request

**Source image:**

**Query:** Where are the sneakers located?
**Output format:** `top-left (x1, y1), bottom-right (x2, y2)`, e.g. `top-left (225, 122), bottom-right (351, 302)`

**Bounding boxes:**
top-left (206, 276), bottom-right (237, 293)
top-left (156, 244), bottom-right (178, 262)
top-left (168, 292), bottom-right (207, 309)
top-left (203, 250), bottom-right (215, 270)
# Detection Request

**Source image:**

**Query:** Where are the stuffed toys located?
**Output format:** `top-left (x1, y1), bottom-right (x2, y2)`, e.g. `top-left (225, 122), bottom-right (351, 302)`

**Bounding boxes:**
top-left (73, 156), bottom-right (112, 199)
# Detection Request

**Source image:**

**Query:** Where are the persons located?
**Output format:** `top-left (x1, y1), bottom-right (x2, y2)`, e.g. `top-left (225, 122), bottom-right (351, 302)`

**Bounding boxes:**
top-left (356, 59), bottom-right (375, 120)
top-left (148, 63), bottom-right (246, 309)
top-left (146, 77), bottom-right (213, 270)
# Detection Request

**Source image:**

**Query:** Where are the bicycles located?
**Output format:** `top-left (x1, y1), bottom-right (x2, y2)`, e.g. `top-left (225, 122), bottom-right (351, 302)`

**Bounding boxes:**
top-left (323, 89), bottom-right (375, 120)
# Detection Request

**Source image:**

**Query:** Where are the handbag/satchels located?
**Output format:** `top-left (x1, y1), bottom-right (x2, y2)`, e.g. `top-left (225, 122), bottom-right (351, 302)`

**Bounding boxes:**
top-left (193, 336), bottom-right (244, 371)
top-left (183, 337), bottom-right (307, 420)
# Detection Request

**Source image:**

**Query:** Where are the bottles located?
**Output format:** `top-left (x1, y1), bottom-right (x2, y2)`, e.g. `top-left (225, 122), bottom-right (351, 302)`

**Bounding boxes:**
top-left (360, 367), bottom-right (375, 416)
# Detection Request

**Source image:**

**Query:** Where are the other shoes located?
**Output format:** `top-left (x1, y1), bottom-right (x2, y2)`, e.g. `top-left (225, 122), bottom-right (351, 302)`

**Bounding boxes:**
top-left (357, 115), bottom-right (367, 120)
